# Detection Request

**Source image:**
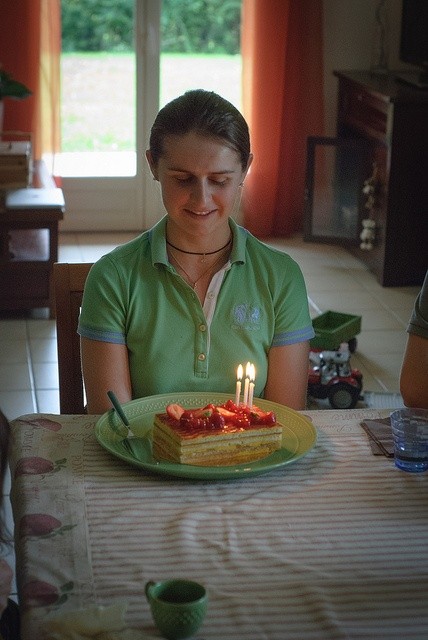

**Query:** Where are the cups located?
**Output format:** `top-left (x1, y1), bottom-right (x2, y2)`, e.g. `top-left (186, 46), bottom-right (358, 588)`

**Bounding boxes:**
top-left (389, 408), bottom-right (428, 472)
top-left (144, 579), bottom-right (208, 640)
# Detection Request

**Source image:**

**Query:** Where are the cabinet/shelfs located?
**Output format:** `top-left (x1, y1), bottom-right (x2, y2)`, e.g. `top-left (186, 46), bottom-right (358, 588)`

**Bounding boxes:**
top-left (303, 69), bottom-right (427, 286)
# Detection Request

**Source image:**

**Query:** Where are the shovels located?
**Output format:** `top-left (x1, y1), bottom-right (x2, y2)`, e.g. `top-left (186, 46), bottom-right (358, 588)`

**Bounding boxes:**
top-left (105, 389), bottom-right (160, 465)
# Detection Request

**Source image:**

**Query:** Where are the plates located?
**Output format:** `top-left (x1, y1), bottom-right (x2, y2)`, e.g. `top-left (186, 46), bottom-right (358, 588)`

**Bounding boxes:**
top-left (94, 391), bottom-right (317, 481)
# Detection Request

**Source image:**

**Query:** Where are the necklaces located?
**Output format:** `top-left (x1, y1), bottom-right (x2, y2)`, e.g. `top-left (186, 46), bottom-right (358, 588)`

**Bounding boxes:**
top-left (166, 239), bottom-right (233, 264)
top-left (167, 247), bottom-right (231, 294)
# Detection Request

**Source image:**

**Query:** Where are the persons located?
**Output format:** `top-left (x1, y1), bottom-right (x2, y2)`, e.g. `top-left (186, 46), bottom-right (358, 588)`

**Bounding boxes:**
top-left (75, 86), bottom-right (315, 415)
top-left (398, 271), bottom-right (428, 408)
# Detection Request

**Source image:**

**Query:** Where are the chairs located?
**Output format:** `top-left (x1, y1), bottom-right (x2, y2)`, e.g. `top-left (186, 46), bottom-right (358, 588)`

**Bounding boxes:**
top-left (52, 263), bottom-right (93, 414)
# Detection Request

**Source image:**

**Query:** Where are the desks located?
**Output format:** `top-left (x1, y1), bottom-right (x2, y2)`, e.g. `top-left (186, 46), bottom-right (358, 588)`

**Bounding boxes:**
top-left (0, 160), bottom-right (65, 319)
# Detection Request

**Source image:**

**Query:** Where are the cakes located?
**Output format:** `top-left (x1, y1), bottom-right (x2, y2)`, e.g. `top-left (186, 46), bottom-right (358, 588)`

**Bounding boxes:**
top-left (151, 400), bottom-right (283, 466)
